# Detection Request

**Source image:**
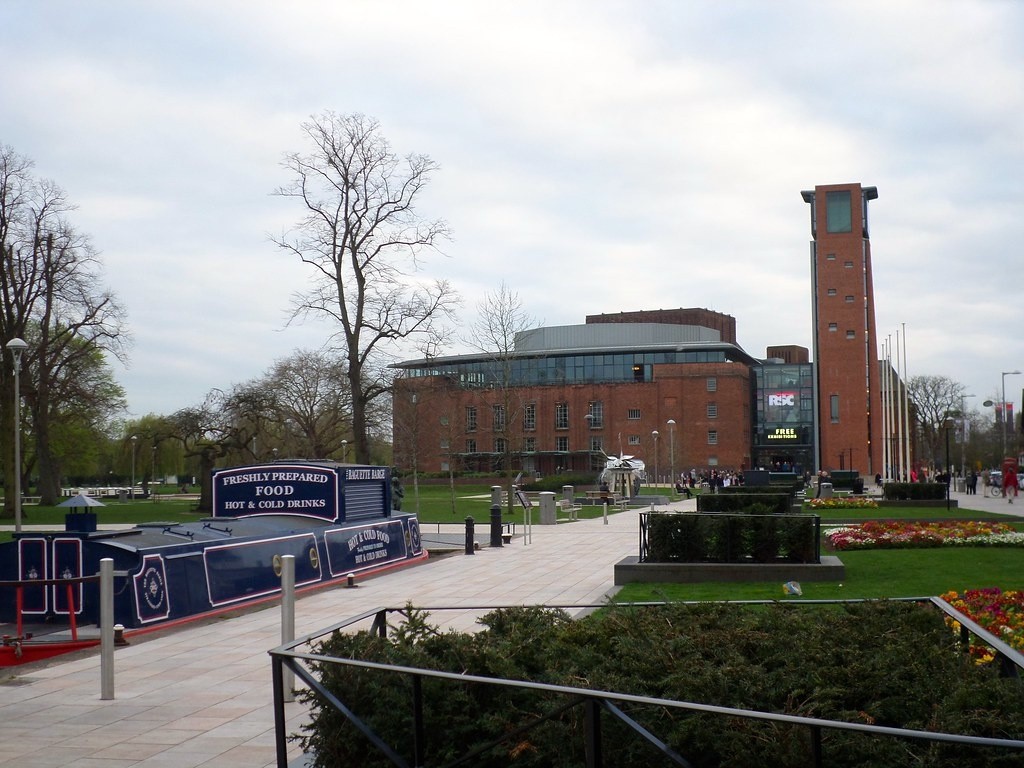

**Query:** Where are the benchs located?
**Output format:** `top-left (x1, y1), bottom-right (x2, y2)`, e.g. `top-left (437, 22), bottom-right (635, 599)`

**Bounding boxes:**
top-left (559, 499), bottom-right (582, 520)
top-left (586, 490), bottom-right (620, 506)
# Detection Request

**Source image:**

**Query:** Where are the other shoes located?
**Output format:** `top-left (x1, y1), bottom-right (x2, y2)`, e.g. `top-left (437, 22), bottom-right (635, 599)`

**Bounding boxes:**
top-left (691, 494), bottom-right (693, 496)
top-left (688, 498), bottom-right (690, 499)
top-left (1008, 499), bottom-right (1013, 504)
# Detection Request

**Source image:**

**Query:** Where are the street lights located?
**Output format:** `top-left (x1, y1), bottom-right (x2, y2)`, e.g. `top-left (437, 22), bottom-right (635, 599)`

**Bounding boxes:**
top-left (272, 448), bottom-right (278, 461)
top-left (961, 393), bottom-right (976, 441)
top-left (652, 430), bottom-right (659, 483)
top-left (5, 338), bottom-right (30, 531)
top-left (252, 436), bottom-right (258, 465)
top-left (340, 439), bottom-right (348, 464)
top-left (944, 416), bottom-right (956, 511)
top-left (1002, 370), bottom-right (1022, 457)
top-left (667, 420), bottom-right (676, 496)
top-left (130, 435), bottom-right (138, 500)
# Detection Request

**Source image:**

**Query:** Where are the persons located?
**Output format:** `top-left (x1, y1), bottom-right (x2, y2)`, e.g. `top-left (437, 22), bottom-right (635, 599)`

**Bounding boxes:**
top-left (965, 471), bottom-right (978, 495)
top-left (392, 477), bottom-right (405, 511)
top-left (803, 471), bottom-right (811, 487)
top-left (815, 470), bottom-right (832, 499)
top-left (600, 481), bottom-right (610, 505)
top-left (875, 473), bottom-right (881, 486)
top-left (768, 461), bottom-right (802, 475)
top-left (681, 468), bottom-right (744, 494)
top-left (1003, 467), bottom-right (1017, 504)
top-left (676, 480), bottom-right (692, 499)
top-left (903, 467), bottom-right (951, 488)
top-left (982, 468), bottom-right (991, 498)
top-left (956, 469), bottom-right (962, 478)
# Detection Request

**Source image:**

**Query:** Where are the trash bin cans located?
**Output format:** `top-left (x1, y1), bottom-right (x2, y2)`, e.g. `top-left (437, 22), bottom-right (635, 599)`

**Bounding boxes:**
top-left (512, 485), bottom-right (519, 504)
top-left (958, 479), bottom-right (965, 492)
top-left (490, 486), bottom-right (502, 506)
top-left (820, 483), bottom-right (832, 498)
top-left (813, 481), bottom-right (819, 498)
top-left (539, 491), bottom-right (557, 524)
top-left (119, 490), bottom-right (127, 503)
top-left (702, 483), bottom-right (709, 494)
top-left (562, 485), bottom-right (573, 504)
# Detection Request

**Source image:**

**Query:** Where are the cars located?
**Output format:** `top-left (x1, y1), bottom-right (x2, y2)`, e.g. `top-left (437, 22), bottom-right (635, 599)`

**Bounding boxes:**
top-left (988, 471), bottom-right (1024, 497)
